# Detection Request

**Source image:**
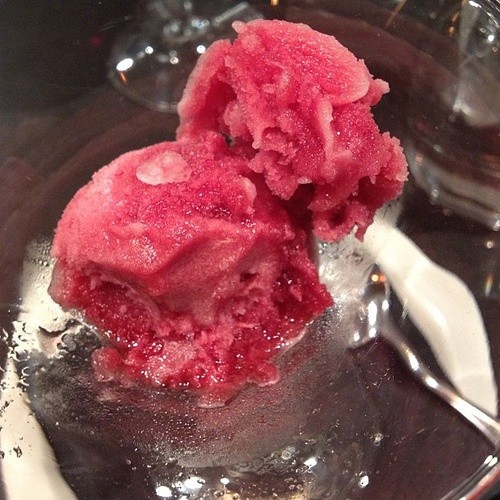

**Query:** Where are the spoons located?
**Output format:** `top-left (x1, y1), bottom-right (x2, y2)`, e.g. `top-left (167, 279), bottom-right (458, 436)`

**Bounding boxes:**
top-left (345, 266), bottom-right (500, 456)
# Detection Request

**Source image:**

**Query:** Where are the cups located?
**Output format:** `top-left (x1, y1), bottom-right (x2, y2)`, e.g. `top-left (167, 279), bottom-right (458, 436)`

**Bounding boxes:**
top-left (405, 0), bottom-right (500, 230)
